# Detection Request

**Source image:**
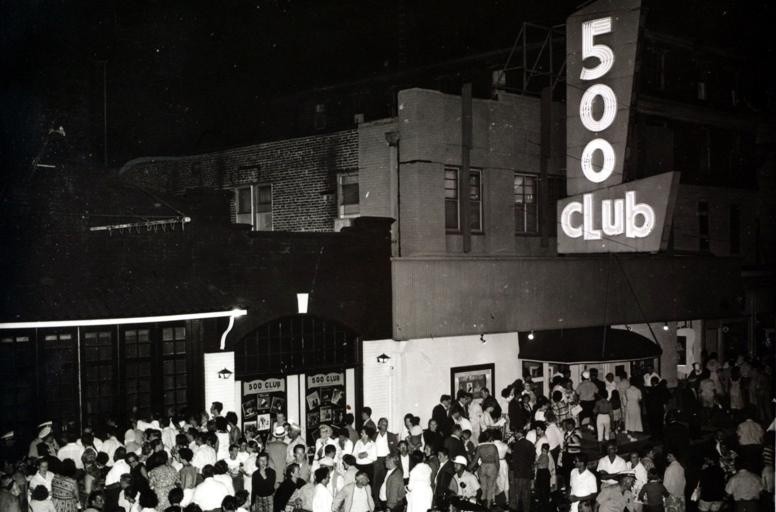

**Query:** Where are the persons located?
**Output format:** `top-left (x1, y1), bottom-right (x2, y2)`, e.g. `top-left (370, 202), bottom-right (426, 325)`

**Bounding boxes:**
top-left (0, 329), bottom-right (776, 512)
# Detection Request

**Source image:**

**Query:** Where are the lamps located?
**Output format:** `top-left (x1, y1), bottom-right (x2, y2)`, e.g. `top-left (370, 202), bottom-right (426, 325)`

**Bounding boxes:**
top-left (377, 353), bottom-right (391, 364)
top-left (218, 367), bottom-right (232, 380)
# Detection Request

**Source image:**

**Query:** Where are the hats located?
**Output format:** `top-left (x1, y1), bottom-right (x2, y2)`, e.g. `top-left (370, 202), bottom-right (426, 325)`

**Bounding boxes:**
top-left (38, 421), bottom-right (54, 441)
top-left (272, 426), bottom-right (288, 437)
top-left (320, 457), bottom-right (335, 466)
top-left (583, 370), bottom-right (590, 379)
top-left (452, 455), bottom-right (468, 466)
top-left (2, 431), bottom-right (15, 441)
top-left (614, 470), bottom-right (637, 479)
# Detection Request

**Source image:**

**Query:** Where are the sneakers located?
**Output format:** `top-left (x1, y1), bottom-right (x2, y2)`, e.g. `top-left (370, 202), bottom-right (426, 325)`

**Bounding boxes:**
top-left (616, 426), bottom-right (640, 442)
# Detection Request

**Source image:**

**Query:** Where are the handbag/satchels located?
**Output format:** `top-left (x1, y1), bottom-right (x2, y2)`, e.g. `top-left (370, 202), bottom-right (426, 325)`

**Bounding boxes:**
top-left (691, 487), bottom-right (701, 501)
top-left (571, 405), bottom-right (583, 416)
